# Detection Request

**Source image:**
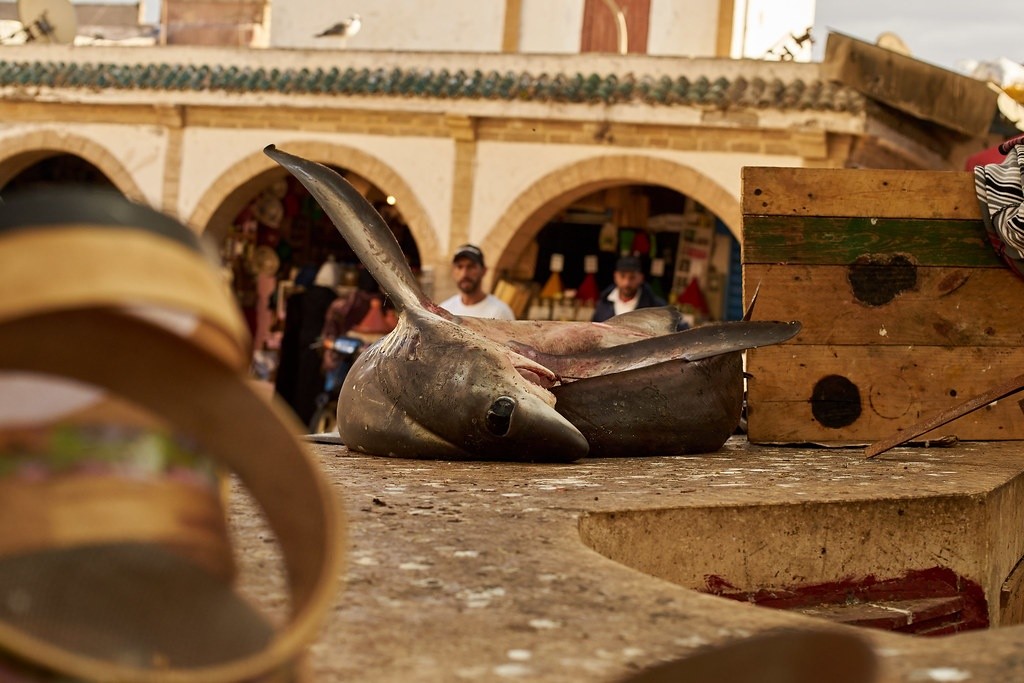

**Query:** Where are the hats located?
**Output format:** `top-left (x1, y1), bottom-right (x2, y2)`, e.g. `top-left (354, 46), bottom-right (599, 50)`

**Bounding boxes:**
top-left (453, 244), bottom-right (484, 266)
top-left (615, 256), bottom-right (644, 274)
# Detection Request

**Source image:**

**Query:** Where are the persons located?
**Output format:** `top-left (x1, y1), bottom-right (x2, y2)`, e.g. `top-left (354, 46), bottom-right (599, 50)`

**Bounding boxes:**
top-left (436, 245), bottom-right (517, 323)
top-left (590, 254), bottom-right (670, 323)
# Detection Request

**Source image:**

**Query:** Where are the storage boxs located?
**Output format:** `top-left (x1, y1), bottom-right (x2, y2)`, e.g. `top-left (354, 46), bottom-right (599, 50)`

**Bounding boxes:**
top-left (737, 160), bottom-right (1024, 443)
